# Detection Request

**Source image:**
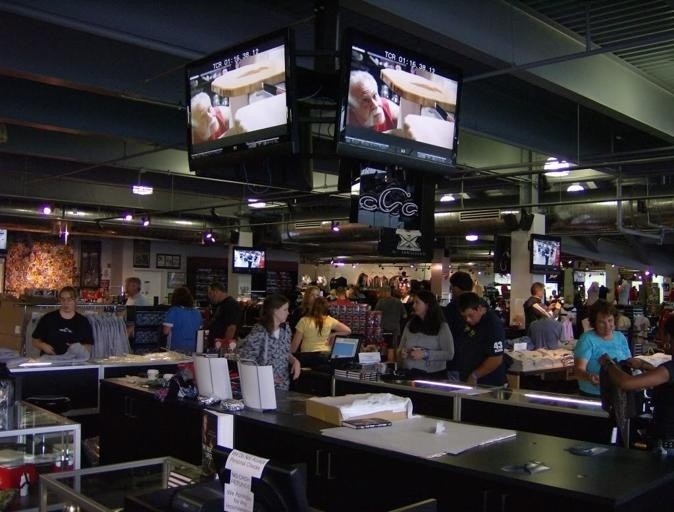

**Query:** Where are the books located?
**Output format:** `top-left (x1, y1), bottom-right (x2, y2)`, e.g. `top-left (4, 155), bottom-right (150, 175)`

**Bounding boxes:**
top-left (201, 408), bottom-right (236, 479)
top-left (167, 466), bottom-right (206, 489)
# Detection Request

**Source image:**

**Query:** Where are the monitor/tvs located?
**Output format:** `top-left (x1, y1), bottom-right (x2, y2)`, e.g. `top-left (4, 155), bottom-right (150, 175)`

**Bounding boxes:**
top-left (232, 245), bottom-right (267, 275)
top-left (196, 101), bottom-right (314, 191)
top-left (184, 26), bottom-right (296, 171)
top-left (494, 236), bottom-right (511, 275)
top-left (528, 234), bottom-right (561, 275)
top-left (334, 38), bottom-right (462, 174)
top-left (328, 335), bottom-right (361, 364)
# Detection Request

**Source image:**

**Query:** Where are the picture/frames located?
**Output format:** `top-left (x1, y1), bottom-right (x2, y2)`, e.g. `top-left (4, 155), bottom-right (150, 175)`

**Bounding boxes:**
top-left (156, 253), bottom-right (181, 269)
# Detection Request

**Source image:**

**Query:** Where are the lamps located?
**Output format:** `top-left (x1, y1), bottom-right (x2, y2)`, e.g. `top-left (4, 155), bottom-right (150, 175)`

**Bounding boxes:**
top-left (200, 228), bottom-right (216, 243)
top-left (141, 215), bottom-right (149, 226)
top-left (567, 183), bottom-right (584, 192)
top-left (122, 212), bottom-right (132, 222)
top-left (133, 185), bottom-right (153, 195)
top-left (331, 220), bottom-right (340, 232)
top-left (466, 235), bottom-right (478, 241)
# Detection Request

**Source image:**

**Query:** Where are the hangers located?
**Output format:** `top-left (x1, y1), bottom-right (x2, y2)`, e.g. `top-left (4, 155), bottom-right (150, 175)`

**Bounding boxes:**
top-left (24, 304), bottom-right (115, 317)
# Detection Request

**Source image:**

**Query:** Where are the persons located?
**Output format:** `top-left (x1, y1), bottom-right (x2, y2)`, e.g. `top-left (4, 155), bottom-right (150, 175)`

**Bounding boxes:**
top-left (191, 92), bottom-right (229, 144)
top-left (123, 276), bottom-right (149, 306)
top-left (238, 250), bottom-right (265, 268)
top-left (201, 415), bottom-right (209, 445)
top-left (346, 70), bottom-right (402, 136)
top-left (537, 240), bottom-right (557, 266)
top-left (162, 287), bottom-right (204, 356)
top-left (237, 269), bottom-right (674, 457)
top-left (31, 285), bottom-right (95, 359)
top-left (207, 280), bottom-right (237, 339)
